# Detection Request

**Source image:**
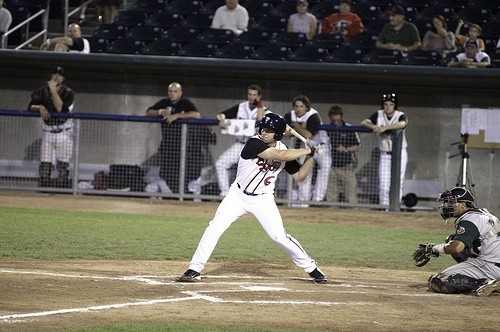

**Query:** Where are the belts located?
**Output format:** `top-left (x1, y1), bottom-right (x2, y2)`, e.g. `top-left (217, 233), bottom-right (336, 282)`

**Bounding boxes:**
top-left (237, 183), bottom-right (266, 197)
top-left (384, 151), bottom-right (392, 155)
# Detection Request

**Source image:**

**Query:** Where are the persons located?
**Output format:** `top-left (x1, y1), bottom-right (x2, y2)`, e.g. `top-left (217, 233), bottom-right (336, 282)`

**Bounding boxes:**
top-left (77, 0), bottom-right (125, 26)
top-left (143, 84), bottom-right (205, 199)
top-left (320, 104), bottom-right (360, 202)
top-left (0, 2), bottom-right (14, 44)
top-left (28, 63), bottom-right (78, 189)
top-left (179, 114), bottom-right (328, 282)
top-left (41, 23), bottom-right (92, 56)
top-left (213, 85), bottom-right (274, 194)
top-left (279, 96), bottom-right (334, 204)
top-left (203, 0), bottom-right (256, 38)
top-left (360, 93), bottom-right (409, 204)
top-left (411, 187), bottom-right (500, 297)
top-left (283, 0), bottom-right (500, 68)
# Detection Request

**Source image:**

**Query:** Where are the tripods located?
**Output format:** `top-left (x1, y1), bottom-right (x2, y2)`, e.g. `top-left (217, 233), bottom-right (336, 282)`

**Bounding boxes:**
top-left (453, 133), bottom-right (477, 207)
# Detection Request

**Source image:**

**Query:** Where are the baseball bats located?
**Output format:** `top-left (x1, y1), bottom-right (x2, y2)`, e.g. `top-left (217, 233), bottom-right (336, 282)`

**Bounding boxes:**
top-left (262, 106), bottom-right (321, 154)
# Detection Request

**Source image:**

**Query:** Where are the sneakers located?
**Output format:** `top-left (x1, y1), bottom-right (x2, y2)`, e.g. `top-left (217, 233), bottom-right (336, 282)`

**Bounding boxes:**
top-left (475, 280), bottom-right (500, 296)
top-left (179, 269), bottom-right (201, 282)
top-left (309, 267), bottom-right (326, 283)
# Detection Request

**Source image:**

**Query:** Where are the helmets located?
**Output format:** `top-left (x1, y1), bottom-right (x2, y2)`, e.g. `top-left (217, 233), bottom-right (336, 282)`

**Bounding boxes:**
top-left (380, 92), bottom-right (399, 109)
top-left (441, 188), bottom-right (478, 208)
top-left (256, 112), bottom-right (287, 141)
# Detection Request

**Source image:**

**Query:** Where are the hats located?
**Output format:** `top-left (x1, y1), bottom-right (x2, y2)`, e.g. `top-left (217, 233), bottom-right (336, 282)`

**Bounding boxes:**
top-left (386, 6), bottom-right (404, 15)
top-left (49, 65), bottom-right (65, 75)
top-left (296, 0), bottom-right (309, 6)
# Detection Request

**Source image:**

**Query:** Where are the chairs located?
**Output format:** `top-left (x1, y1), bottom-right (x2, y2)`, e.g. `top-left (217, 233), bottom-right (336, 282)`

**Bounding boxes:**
top-left (0, 1), bottom-right (500, 68)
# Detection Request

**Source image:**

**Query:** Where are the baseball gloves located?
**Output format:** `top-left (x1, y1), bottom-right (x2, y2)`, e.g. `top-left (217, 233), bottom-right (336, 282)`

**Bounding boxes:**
top-left (412, 244), bottom-right (439, 267)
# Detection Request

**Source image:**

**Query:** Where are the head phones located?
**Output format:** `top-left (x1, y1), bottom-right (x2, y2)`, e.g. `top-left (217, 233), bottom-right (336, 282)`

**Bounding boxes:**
top-left (258, 120), bottom-right (286, 141)
